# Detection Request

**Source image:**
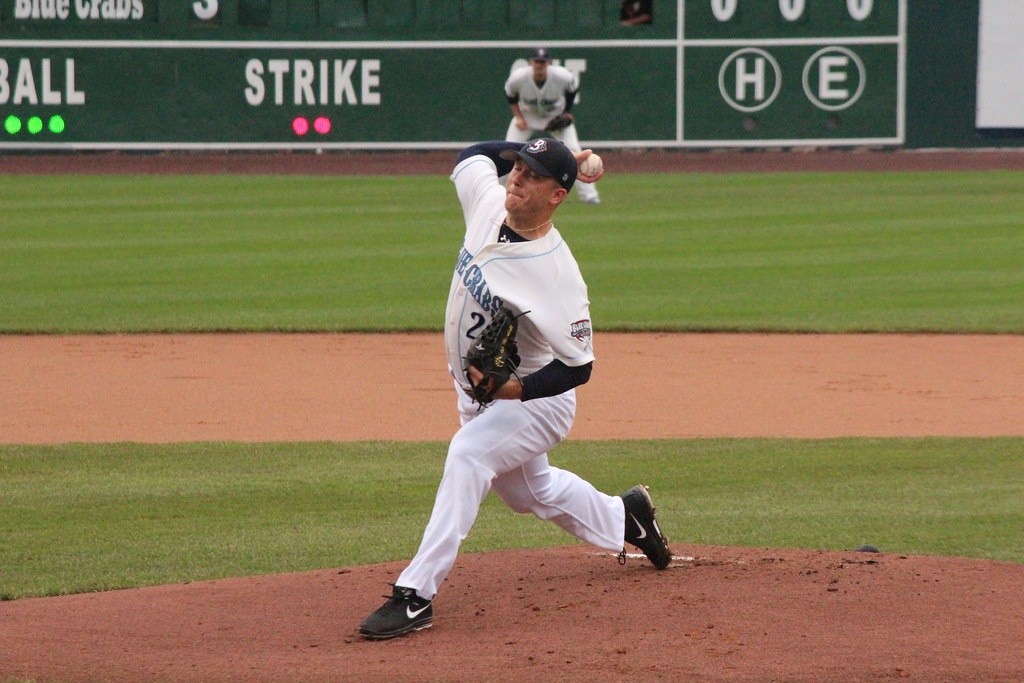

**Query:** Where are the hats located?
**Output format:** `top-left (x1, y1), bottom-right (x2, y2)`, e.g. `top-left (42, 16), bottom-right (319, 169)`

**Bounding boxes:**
top-left (499, 134), bottom-right (580, 195)
top-left (525, 50), bottom-right (556, 64)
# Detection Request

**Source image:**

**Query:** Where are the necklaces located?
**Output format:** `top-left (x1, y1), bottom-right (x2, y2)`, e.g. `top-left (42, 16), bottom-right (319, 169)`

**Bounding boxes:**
top-left (504, 218), bottom-right (552, 232)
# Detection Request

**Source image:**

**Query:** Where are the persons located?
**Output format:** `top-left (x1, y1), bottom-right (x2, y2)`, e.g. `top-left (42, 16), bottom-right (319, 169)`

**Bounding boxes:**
top-left (357, 136), bottom-right (671, 639)
top-left (505, 47), bottom-right (600, 203)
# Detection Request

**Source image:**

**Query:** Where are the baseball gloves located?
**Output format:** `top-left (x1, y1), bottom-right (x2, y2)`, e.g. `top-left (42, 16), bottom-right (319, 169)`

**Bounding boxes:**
top-left (543, 113), bottom-right (574, 134)
top-left (462, 304), bottom-right (531, 406)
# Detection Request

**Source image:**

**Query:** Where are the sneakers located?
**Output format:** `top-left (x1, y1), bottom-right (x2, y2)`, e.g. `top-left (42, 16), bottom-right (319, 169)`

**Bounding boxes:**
top-left (587, 186), bottom-right (605, 208)
top-left (624, 479), bottom-right (674, 579)
top-left (353, 582), bottom-right (445, 640)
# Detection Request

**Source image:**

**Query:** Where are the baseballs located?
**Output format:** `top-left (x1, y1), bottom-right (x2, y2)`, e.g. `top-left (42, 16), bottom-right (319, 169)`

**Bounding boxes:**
top-left (579, 153), bottom-right (604, 177)
